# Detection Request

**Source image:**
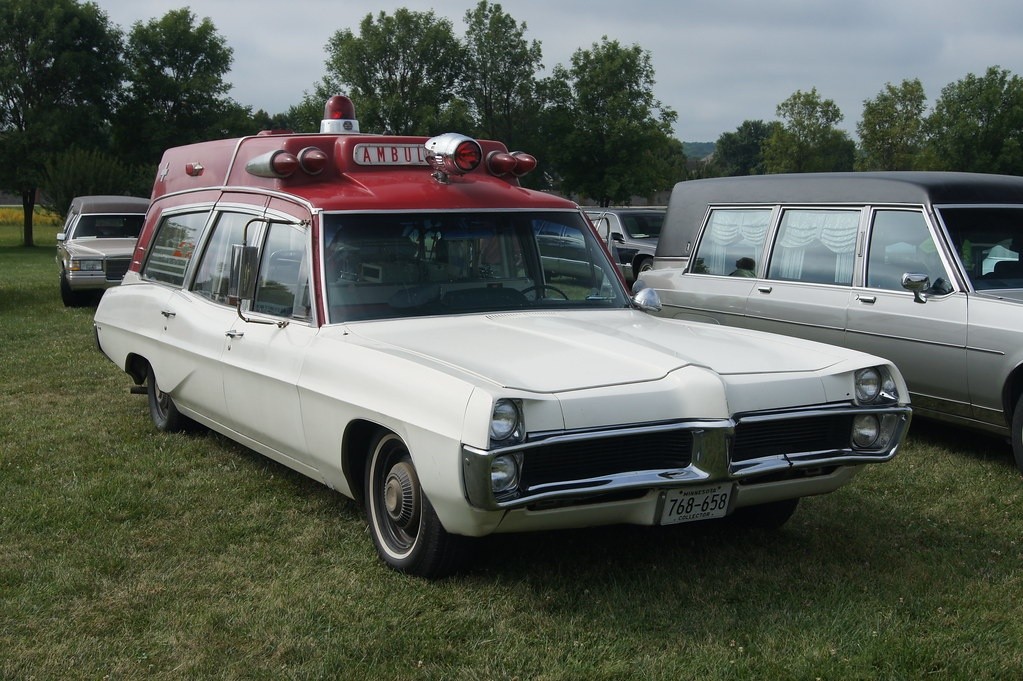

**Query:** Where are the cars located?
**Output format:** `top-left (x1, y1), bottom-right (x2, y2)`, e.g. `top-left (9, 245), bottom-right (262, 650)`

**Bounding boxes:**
top-left (56, 171), bottom-right (1023, 466)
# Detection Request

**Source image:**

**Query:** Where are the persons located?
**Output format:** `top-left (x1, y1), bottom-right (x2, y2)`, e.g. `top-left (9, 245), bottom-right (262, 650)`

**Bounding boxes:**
top-left (729, 257), bottom-right (756, 279)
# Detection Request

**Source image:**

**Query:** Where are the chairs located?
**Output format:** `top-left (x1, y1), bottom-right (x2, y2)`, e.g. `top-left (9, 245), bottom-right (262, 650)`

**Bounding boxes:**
top-left (625, 220), bottom-right (640, 234)
top-left (119, 218), bottom-right (140, 236)
top-left (77, 217), bottom-right (100, 236)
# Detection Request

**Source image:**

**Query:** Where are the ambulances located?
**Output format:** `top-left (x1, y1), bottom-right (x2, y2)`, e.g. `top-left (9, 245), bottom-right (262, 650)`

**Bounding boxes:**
top-left (94, 95), bottom-right (912, 575)
